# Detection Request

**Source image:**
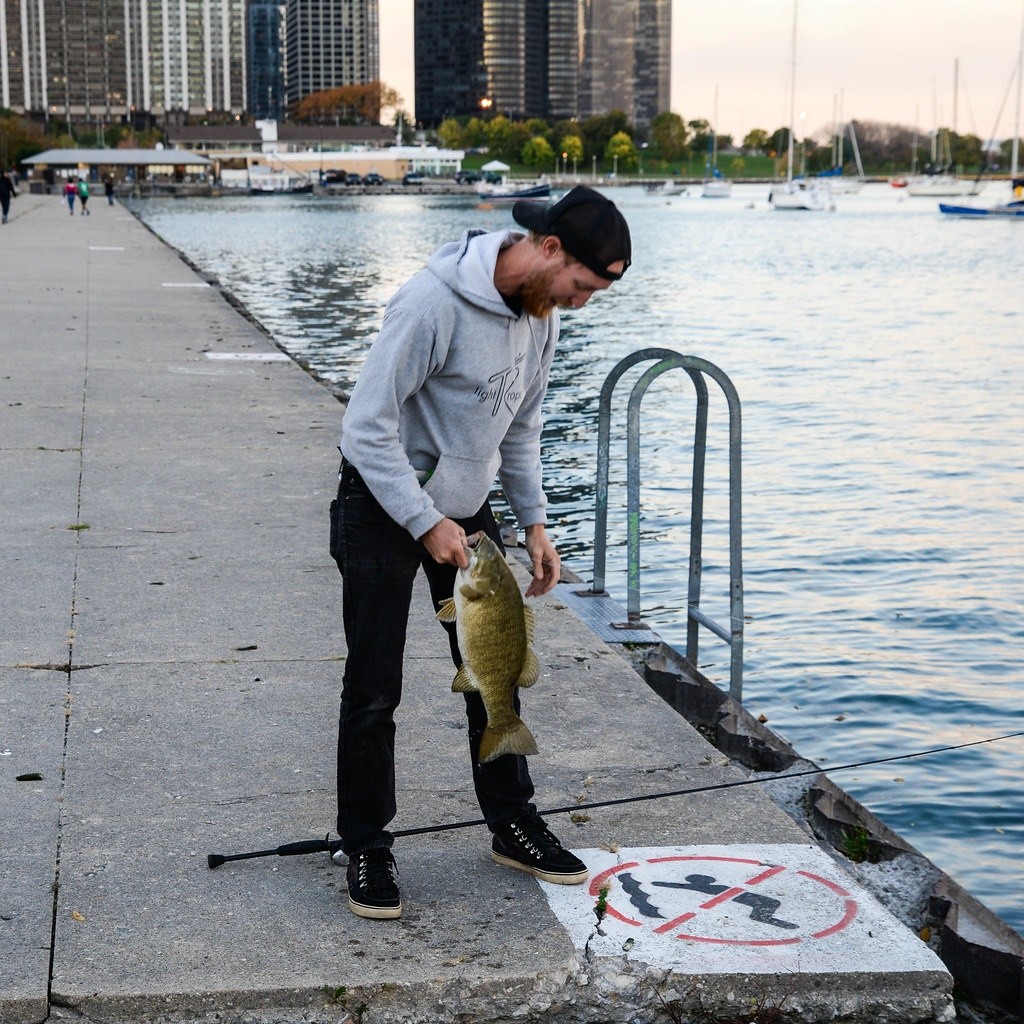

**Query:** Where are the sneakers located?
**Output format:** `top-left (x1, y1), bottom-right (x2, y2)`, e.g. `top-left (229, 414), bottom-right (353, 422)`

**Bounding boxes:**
top-left (489, 816), bottom-right (590, 885)
top-left (344, 843), bottom-right (402, 920)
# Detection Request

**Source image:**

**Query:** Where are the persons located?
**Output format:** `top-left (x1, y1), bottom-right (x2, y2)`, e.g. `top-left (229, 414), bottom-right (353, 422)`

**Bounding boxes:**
top-left (0, 170), bottom-right (19, 223)
top-left (105, 178), bottom-right (114, 205)
top-left (63, 177), bottom-right (90, 215)
top-left (329, 186), bottom-right (631, 918)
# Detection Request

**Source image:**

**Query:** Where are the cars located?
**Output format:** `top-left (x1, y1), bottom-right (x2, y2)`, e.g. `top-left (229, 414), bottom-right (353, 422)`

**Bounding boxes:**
top-left (363, 173), bottom-right (384, 186)
top-left (482, 171), bottom-right (503, 186)
top-left (454, 171), bottom-right (481, 185)
top-left (403, 173), bottom-right (423, 186)
top-left (344, 173), bottom-right (362, 186)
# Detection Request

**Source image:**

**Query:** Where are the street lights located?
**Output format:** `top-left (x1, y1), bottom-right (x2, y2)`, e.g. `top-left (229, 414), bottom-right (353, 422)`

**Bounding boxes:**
top-left (638, 142), bottom-right (648, 178)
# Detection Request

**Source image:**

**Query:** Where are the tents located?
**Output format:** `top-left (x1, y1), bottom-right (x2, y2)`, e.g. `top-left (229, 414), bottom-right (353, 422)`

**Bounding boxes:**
top-left (481, 160), bottom-right (510, 185)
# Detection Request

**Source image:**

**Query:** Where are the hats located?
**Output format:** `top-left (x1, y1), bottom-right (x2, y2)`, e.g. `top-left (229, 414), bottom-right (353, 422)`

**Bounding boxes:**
top-left (512, 183), bottom-right (632, 282)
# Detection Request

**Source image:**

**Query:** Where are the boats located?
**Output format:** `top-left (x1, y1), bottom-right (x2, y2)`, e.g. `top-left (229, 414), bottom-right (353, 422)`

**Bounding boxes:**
top-left (644, 179), bottom-right (684, 197)
top-left (700, 83), bottom-right (733, 198)
top-left (814, 36), bottom-right (1024, 219)
top-left (247, 152), bottom-right (314, 198)
top-left (769, 0), bottom-right (832, 210)
top-left (474, 177), bottom-right (552, 202)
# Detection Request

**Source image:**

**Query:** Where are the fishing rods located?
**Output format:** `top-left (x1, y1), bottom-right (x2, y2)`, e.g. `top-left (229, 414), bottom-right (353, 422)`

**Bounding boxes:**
top-left (204, 727), bottom-right (1023, 870)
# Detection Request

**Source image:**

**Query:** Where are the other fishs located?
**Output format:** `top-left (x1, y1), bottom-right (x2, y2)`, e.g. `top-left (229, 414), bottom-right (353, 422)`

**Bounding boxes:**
top-left (435, 530), bottom-right (540, 762)
